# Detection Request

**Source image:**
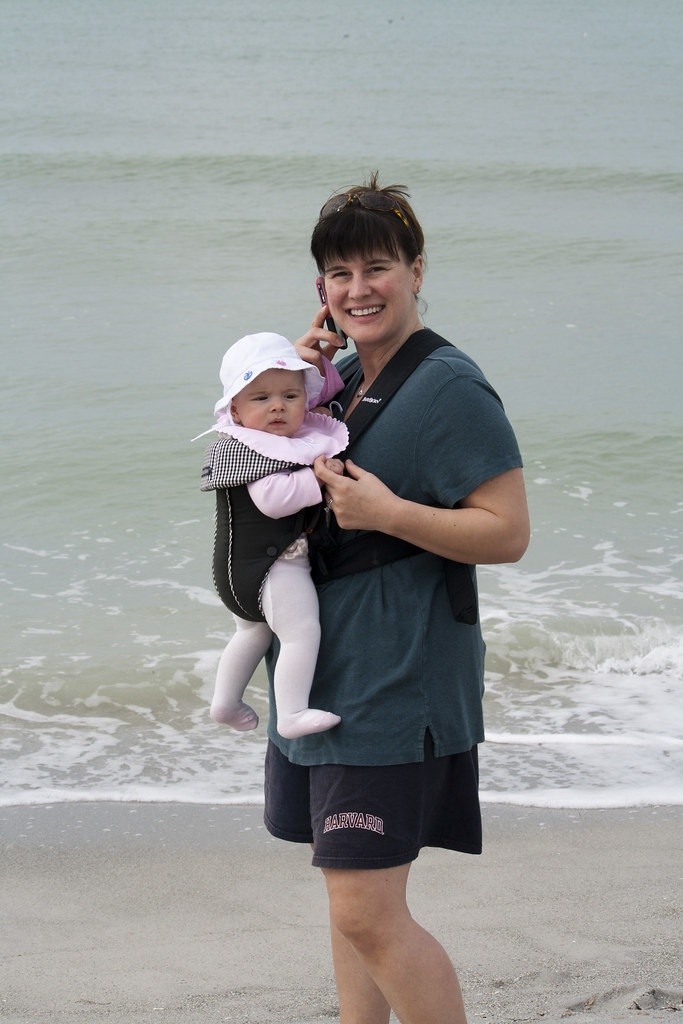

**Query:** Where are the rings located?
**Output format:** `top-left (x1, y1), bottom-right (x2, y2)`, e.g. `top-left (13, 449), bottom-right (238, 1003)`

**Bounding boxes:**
top-left (323, 498), bottom-right (334, 513)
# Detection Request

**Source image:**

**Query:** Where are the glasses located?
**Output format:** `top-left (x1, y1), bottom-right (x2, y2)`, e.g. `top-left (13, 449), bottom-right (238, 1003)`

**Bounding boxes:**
top-left (318, 192), bottom-right (420, 254)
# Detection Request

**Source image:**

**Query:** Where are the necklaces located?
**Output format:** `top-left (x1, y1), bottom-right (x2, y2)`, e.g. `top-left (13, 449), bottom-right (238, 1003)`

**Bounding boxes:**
top-left (355, 387), bottom-right (364, 398)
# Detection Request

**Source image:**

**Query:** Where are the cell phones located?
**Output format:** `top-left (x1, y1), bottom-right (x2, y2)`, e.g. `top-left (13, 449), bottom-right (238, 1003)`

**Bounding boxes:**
top-left (316, 276), bottom-right (348, 350)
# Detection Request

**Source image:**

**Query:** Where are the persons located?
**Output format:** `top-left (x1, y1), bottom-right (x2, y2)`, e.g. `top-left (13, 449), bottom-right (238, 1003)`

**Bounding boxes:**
top-left (192, 331), bottom-right (350, 741)
top-left (262, 170), bottom-right (532, 1023)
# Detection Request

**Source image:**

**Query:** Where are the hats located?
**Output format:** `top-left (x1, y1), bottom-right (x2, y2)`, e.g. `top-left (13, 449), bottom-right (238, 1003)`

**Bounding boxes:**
top-left (213, 332), bottom-right (325, 419)
